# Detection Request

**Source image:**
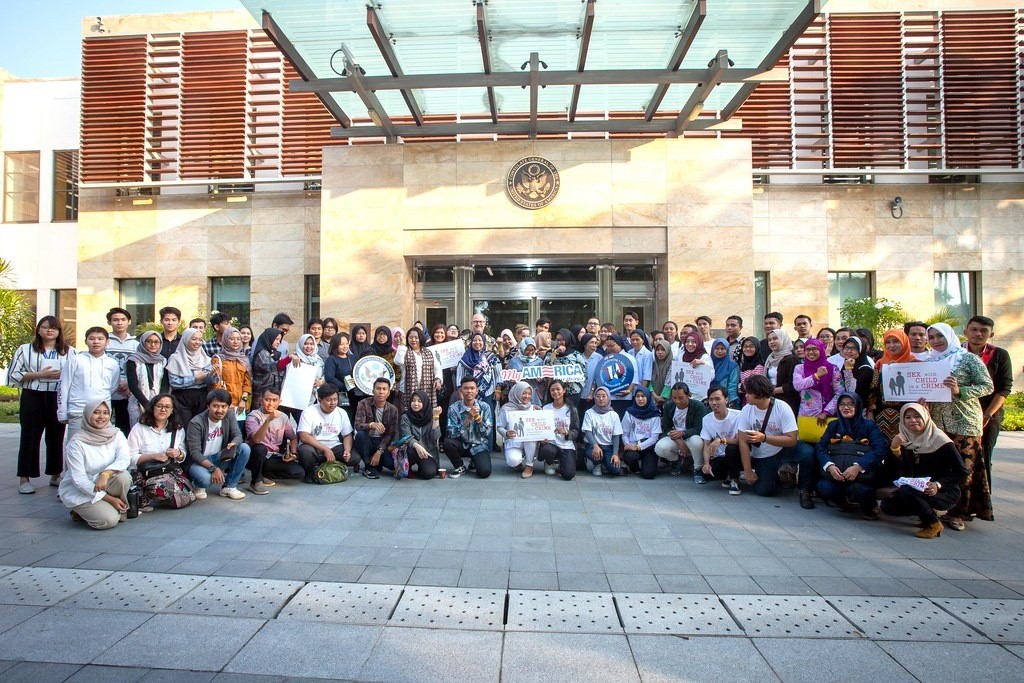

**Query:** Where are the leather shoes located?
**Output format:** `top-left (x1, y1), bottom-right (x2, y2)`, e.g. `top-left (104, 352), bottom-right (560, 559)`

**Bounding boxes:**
top-left (940, 513), bottom-right (965, 531)
top-left (514, 464), bottom-right (533, 478)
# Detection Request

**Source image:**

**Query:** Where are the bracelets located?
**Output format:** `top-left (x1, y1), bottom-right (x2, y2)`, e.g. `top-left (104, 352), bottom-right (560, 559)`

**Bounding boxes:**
top-left (369, 422), bottom-right (374, 428)
top-left (102, 472), bottom-right (110, 479)
top-left (845, 366), bottom-right (854, 370)
top-left (432, 415), bottom-right (439, 418)
top-left (762, 434), bottom-right (766, 443)
top-left (292, 453), bottom-right (298, 457)
top-left (637, 445), bottom-right (640, 451)
top-left (209, 466), bottom-right (217, 473)
top-left (474, 415), bottom-right (481, 420)
top-left (554, 349), bottom-right (559, 355)
top-left (495, 390), bottom-right (501, 393)
top-left (890, 445), bottom-right (901, 451)
top-left (565, 430), bottom-right (568, 435)
top-left (720, 437), bottom-right (727, 445)
top-left (936, 481), bottom-right (942, 490)
top-left (242, 394), bottom-right (248, 398)
top-left (814, 373), bottom-right (820, 381)
top-left (498, 348), bottom-right (502, 352)
top-left (175, 449), bottom-right (183, 459)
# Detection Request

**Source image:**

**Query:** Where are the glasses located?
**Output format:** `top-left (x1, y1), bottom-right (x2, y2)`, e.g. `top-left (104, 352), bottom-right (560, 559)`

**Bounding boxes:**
top-left (40, 325), bottom-right (58, 329)
top-left (473, 321), bottom-right (485, 324)
top-left (839, 402), bottom-right (855, 408)
top-left (324, 326), bottom-right (335, 330)
top-left (154, 405), bottom-right (171, 410)
top-left (587, 322), bottom-right (599, 326)
top-left (805, 334), bottom-right (857, 352)
top-left (280, 327), bottom-right (290, 333)
top-left (146, 340), bottom-right (160, 344)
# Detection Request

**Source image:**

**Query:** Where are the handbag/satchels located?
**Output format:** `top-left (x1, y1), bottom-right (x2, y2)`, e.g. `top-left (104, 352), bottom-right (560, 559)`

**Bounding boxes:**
top-left (314, 461), bottom-right (348, 485)
top-left (347, 387), bottom-right (368, 408)
top-left (392, 444), bottom-right (409, 480)
top-left (207, 355), bottom-right (227, 392)
top-left (139, 458), bottom-right (173, 479)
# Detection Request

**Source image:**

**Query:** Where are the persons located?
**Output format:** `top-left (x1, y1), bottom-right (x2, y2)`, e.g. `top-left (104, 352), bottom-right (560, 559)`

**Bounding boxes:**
top-left (9, 307), bottom-right (1011, 538)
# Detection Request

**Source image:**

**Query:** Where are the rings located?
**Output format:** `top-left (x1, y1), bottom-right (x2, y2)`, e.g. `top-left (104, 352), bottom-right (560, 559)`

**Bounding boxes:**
top-left (833, 477), bottom-right (835, 479)
top-left (931, 489), bottom-right (934, 493)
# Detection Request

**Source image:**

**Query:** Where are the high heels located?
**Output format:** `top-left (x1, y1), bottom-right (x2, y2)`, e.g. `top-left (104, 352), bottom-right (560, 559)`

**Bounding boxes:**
top-left (915, 521), bottom-right (944, 538)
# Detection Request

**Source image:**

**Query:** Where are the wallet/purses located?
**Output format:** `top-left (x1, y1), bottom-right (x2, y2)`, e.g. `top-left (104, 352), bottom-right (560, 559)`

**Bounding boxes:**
top-left (219, 444), bottom-right (236, 462)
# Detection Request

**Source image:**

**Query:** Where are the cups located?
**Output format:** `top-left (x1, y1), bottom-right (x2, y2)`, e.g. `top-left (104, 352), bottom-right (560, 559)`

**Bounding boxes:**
top-left (746, 430), bottom-right (756, 441)
top-left (438, 469), bottom-right (446, 479)
top-left (670, 430), bottom-right (676, 437)
top-left (354, 465), bottom-right (360, 473)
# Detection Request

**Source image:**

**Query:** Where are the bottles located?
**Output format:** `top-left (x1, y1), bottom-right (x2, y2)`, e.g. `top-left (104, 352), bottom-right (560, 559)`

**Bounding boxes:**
top-left (285, 439), bottom-right (290, 460)
top-left (127, 486), bottom-right (139, 519)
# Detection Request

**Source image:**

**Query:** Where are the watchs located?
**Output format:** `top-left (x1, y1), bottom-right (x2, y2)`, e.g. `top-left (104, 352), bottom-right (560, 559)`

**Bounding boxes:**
top-left (984, 410), bottom-right (992, 420)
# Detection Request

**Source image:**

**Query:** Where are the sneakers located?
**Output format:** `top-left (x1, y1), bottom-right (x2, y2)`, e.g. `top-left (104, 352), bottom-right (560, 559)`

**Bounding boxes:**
top-left (449, 458), bottom-right (477, 478)
top-left (363, 466), bottom-right (383, 479)
top-left (722, 470), bottom-right (882, 520)
top-left (587, 459), bottom-right (707, 484)
top-left (194, 473), bottom-right (315, 500)
top-left (544, 462), bottom-right (556, 476)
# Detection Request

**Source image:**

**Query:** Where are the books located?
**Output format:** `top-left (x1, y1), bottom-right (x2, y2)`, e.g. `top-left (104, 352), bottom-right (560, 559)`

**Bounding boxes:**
top-left (40, 359), bottom-right (60, 383)
top-left (235, 406), bottom-right (246, 421)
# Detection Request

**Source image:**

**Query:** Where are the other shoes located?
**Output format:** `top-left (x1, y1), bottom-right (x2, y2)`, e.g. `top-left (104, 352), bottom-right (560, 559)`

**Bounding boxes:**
top-left (440, 444), bottom-right (445, 453)
top-left (70, 510), bottom-right (79, 521)
top-left (18, 476), bottom-right (63, 494)
top-left (119, 495), bottom-right (153, 522)
top-left (493, 442), bottom-right (501, 452)
